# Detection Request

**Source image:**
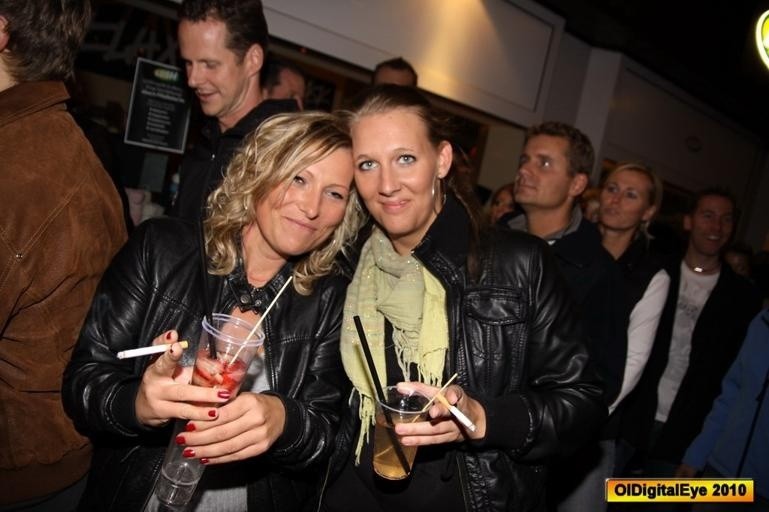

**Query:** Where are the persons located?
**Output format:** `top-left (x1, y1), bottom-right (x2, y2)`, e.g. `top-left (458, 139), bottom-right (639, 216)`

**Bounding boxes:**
top-left (0, 0), bottom-right (767, 512)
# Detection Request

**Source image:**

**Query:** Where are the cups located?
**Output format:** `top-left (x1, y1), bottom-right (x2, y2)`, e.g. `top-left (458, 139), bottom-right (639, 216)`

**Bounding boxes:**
top-left (188, 312), bottom-right (266, 420)
top-left (373, 386), bottom-right (435, 482)
